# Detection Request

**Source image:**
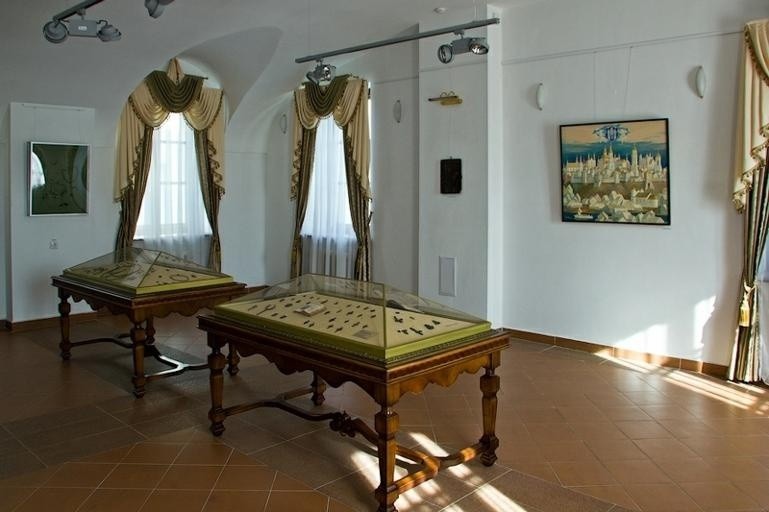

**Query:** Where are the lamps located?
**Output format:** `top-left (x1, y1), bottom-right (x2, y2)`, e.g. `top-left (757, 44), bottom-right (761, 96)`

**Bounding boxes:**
top-left (43, 0), bottom-right (171, 43)
top-left (293, 17), bottom-right (500, 88)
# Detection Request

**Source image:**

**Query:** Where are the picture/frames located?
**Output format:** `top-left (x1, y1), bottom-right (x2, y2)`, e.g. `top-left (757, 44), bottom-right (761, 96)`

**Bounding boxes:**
top-left (27, 141), bottom-right (93, 216)
top-left (559, 118), bottom-right (671, 224)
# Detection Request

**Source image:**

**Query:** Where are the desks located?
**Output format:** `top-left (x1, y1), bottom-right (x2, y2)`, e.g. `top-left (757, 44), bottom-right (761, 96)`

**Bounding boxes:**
top-left (195, 274), bottom-right (512, 512)
top-left (50, 247), bottom-right (247, 399)
